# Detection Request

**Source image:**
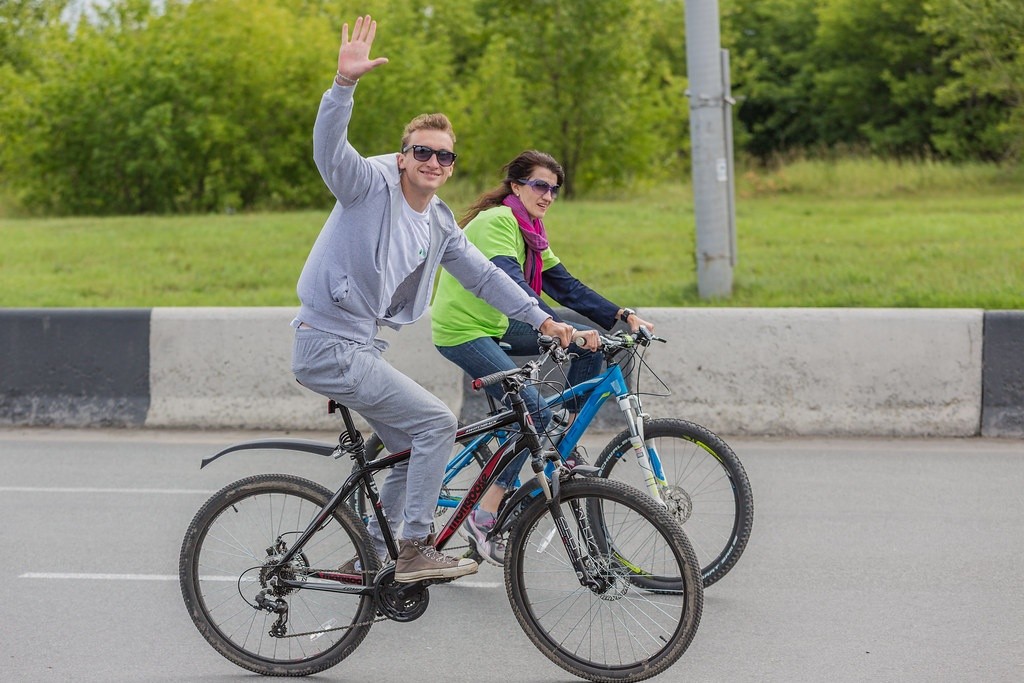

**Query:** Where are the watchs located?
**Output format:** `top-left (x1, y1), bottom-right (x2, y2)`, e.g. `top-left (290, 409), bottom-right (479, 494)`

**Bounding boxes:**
top-left (621, 308), bottom-right (635, 323)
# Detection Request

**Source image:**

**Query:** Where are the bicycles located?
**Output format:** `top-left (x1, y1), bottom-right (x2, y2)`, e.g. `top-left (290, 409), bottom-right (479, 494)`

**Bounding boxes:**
top-left (347, 323), bottom-right (755, 595)
top-left (178, 333), bottom-right (705, 683)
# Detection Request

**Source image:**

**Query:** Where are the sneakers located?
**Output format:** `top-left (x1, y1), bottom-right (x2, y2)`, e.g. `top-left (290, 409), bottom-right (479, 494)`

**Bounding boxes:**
top-left (337, 556), bottom-right (361, 585)
top-left (530, 446), bottom-right (590, 475)
top-left (394, 532), bottom-right (478, 582)
top-left (458, 505), bottom-right (505, 567)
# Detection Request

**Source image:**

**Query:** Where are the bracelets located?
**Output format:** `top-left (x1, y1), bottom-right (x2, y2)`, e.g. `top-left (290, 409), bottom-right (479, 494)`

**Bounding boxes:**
top-left (571, 328), bottom-right (577, 336)
top-left (337, 70), bottom-right (359, 83)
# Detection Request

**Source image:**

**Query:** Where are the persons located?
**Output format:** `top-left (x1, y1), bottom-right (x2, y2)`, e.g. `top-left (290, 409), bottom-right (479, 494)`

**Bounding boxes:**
top-left (291, 15), bottom-right (573, 582)
top-left (431, 150), bottom-right (653, 567)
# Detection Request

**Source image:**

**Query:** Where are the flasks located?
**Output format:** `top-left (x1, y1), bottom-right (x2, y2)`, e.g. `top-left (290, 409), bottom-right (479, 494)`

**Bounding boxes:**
top-left (538, 408), bottom-right (570, 456)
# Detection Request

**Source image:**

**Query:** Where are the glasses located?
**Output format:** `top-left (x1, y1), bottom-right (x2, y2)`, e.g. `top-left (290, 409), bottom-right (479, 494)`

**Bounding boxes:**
top-left (403, 144), bottom-right (457, 167)
top-left (517, 179), bottom-right (560, 199)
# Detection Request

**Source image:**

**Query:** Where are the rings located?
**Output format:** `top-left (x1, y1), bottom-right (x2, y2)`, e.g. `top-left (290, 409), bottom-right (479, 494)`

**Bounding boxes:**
top-left (597, 339), bottom-right (602, 342)
